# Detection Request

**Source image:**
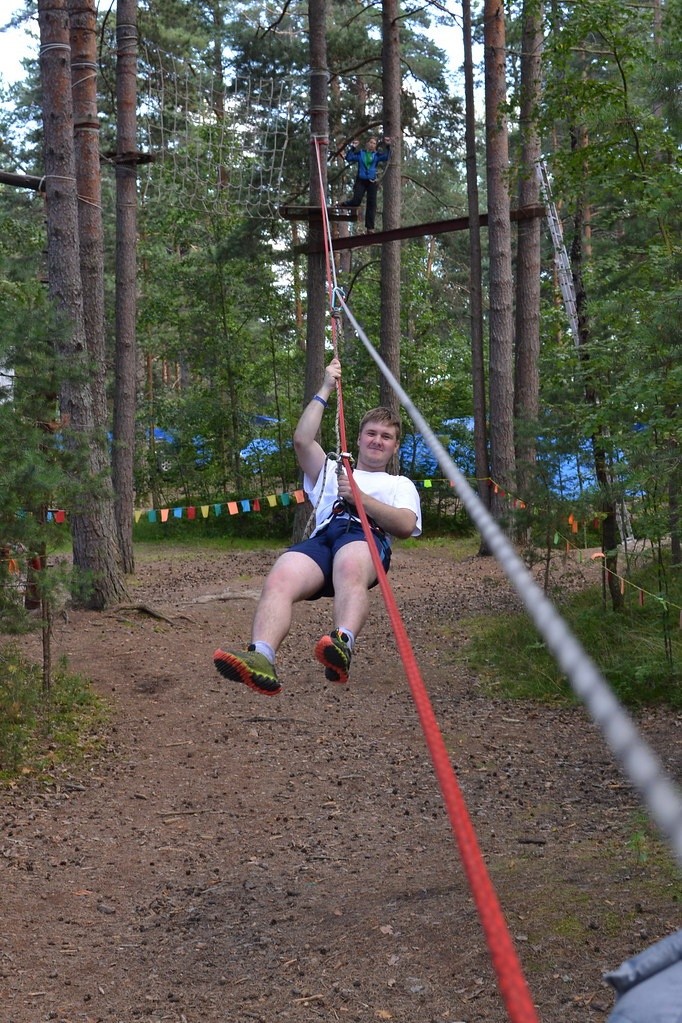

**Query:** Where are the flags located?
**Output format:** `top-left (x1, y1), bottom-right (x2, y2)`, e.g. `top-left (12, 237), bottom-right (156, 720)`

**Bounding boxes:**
top-left (134, 509), bottom-right (141, 523)
top-left (267, 495), bottom-right (277, 507)
top-left (227, 502), bottom-right (238, 515)
top-left (281, 493), bottom-right (289, 506)
top-left (214, 503), bottom-right (221, 517)
top-left (174, 507), bottom-right (182, 518)
top-left (423, 480), bottom-right (432, 488)
top-left (591, 552), bottom-right (605, 561)
top-left (295, 489), bottom-right (304, 503)
top-left (241, 500), bottom-right (251, 512)
top-left (149, 509), bottom-right (156, 523)
top-left (202, 506), bottom-right (209, 518)
top-left (416, 479), bottom-right (424, 487)
top-left (253, 498), bottom-right (260, 510)
top-left (494, 485), bottom-right (582, 564)
top-left (161, 509), bottom-right (168, 522)
top-left (188, 507), bottom-right (195, 519)
top-left (621, 577), bottom-right (625, 594)
top-left (56, 510), bottom-right (66, 523)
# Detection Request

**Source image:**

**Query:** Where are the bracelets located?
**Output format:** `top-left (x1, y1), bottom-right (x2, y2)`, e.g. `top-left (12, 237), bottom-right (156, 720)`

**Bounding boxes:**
top-left (313, 394), bottom-right (328, 408)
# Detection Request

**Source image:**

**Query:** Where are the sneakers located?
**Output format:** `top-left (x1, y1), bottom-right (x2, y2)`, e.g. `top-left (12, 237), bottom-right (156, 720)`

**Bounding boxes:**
top-left (213, 642), bottom-right (282, 696)
top-left (315, 627), bottom-right (352, 685)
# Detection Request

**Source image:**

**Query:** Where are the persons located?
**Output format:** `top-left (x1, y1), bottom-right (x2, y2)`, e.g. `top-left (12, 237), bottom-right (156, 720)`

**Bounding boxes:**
top-left (214, 358), bottom-right (423, 695)
